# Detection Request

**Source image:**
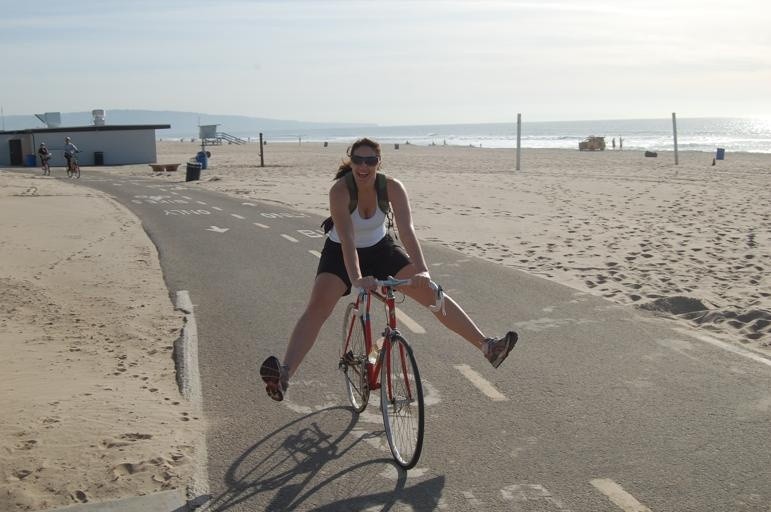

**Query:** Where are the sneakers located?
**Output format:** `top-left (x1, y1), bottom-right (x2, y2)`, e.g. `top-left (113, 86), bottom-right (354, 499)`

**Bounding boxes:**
top-left (485, 331), bottom-right (518, 368)
top-left (260, 356), bottom-right (289, 401)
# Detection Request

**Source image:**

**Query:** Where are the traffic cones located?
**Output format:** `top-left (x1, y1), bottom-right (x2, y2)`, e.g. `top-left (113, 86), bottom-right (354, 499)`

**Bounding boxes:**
top-left (711, 158), bottom-right (716, 165)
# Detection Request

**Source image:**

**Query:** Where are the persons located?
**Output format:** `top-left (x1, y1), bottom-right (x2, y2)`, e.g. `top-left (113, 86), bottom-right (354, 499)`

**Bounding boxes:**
top-left (64, 137), bottom-right (81, 175)
top-left (37, 142), bottom-right (51, 171)
top-left (260, 137), bottom-right (519, 402)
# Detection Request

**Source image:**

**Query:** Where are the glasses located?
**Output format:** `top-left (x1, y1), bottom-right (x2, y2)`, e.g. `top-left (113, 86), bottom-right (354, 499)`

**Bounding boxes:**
top-left (351, 155), bottom-right (378, 166)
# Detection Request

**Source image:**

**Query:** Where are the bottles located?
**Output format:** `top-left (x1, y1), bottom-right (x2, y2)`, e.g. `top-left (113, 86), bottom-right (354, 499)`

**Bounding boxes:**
top-left (367, 331), bottom-right (386, 365)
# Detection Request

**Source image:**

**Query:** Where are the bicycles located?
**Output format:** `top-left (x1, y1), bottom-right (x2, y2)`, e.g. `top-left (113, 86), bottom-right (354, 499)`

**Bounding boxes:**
top-left (339, 275), bottom-right (446, 467)
top-left (64, 150), bottom-right (83, 179)
top-left (38, 152), bottom-right (54, 175)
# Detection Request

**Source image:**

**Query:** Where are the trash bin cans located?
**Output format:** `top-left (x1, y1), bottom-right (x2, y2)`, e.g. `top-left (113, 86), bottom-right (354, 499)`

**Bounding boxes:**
top-left (26, 154), bottom-right (36, 167)
top-left (717, 148), bottom-right (725, 160)
top-left (196, 151), bottom-right (208, 169)
top-left (95, 152), bottom-right (104, 165)
top-left (186, 161), bottom-right (202, 182)
